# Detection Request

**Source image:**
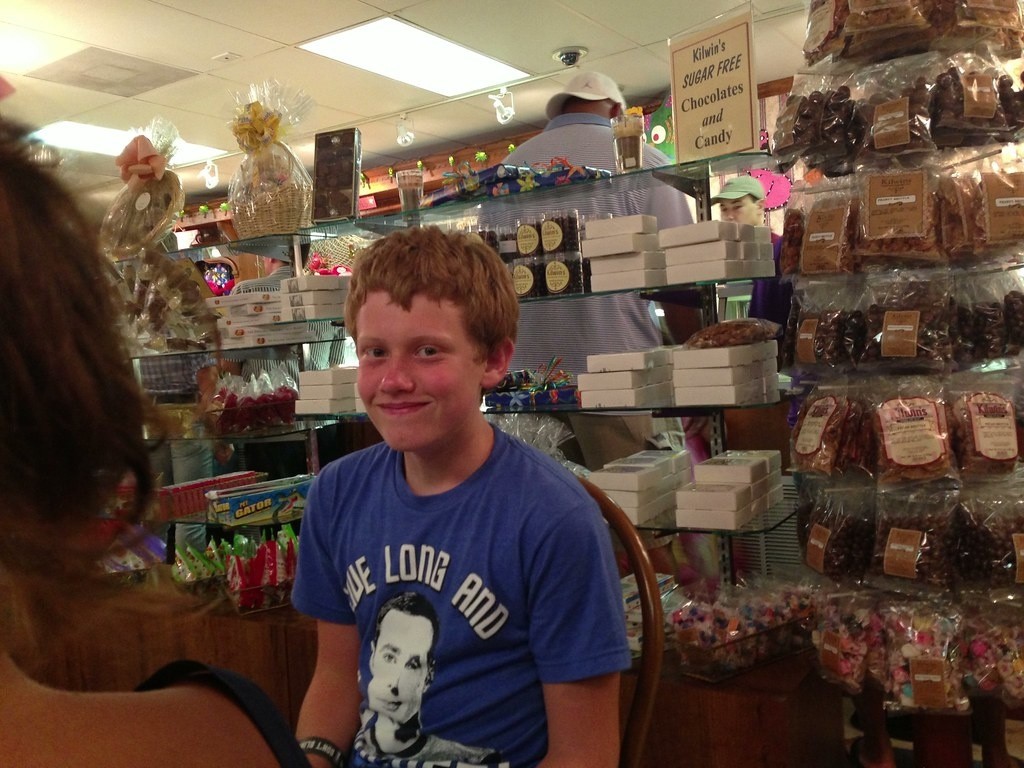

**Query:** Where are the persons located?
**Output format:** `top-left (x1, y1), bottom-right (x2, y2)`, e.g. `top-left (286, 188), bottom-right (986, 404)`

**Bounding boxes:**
top-left (1, 115), bottom-right (306, 768)
top-left (710, 174), bottom-right (794, 323)
top-left (350, 591), bottom-right (497, 765)
top-left (476, 72), bottom-right (708, 471)
top-left (136, 351), bottom-right (218, 565)
top-left (210, 237), bottom-right (347, 484)
top-left (289, 228), bottom-right (635, 768)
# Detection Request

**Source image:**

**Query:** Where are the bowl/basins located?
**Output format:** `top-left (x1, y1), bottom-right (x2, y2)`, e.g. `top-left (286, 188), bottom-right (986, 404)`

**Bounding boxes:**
top-left (174, 230), bottom-right (198, 250)
top-left (217, 219), bottom-right (238, 242)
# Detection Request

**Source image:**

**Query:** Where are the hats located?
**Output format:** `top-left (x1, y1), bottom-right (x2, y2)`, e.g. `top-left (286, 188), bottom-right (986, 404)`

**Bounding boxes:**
top-left (709, 175), bottom-right (765, 206)
top-left (544, 69), bottom-right (627, 121)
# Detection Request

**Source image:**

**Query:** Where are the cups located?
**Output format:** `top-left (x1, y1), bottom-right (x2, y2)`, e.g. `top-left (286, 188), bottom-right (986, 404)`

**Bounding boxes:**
top-left (195, 225), bottom-right (218, 244)
top-left (610, 114), bottom-right (645, 176)
top-left (396, 169), bottom-right (423, 213)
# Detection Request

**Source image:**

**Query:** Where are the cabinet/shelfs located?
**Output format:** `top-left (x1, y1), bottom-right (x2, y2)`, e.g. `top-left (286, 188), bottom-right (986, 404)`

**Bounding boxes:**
top-left (56, 150), bottom-right (844, 768)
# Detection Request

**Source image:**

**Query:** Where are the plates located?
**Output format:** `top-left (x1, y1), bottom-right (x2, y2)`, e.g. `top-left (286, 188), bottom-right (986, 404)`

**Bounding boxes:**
top-left (192, 242), bottom-right (216, 247)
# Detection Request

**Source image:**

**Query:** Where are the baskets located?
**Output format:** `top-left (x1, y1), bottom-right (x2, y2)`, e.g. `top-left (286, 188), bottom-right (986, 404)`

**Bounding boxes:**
top-left (227, 141), bottom-right (315, 243)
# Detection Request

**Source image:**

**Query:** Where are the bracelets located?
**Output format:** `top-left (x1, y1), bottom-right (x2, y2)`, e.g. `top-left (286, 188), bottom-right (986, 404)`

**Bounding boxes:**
top-left (300, 736), bottom-right (345, 768)
